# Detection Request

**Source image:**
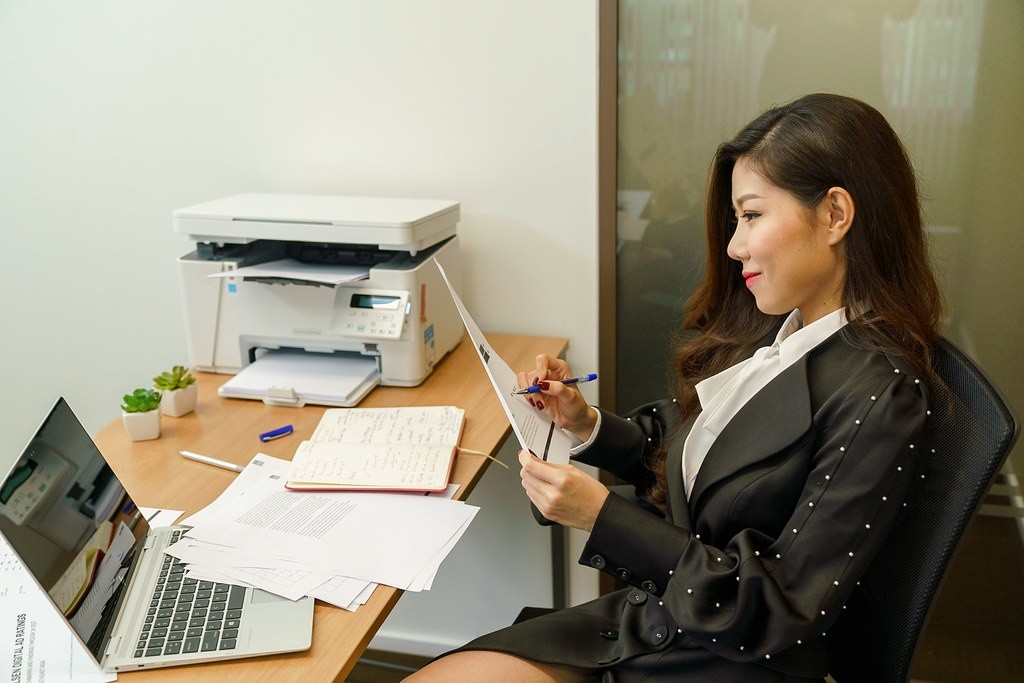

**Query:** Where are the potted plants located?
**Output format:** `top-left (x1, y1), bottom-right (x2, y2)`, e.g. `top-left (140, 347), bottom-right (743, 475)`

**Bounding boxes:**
top-left (121, 389), bottom-right (162, 442)
top-left (152, 365), bottom-right (198, 417)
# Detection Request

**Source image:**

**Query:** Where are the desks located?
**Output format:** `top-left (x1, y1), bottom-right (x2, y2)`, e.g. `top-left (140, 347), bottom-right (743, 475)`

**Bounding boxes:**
top-left (94, 331), bottom-right (570, 683)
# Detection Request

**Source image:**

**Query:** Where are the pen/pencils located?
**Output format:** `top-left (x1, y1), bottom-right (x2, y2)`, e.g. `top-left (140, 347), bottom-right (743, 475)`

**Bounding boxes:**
top-left (513, 374), bottom-right (598, 394)
top-left (178, 451), bottom-right (244, 472)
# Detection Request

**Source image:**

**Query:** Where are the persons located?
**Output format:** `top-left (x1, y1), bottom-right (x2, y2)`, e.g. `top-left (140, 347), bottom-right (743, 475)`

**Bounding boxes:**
top-left (387, 92), bottom-right (950, 683)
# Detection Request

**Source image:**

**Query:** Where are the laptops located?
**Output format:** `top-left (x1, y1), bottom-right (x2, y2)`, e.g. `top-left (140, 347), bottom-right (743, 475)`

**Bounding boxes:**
top-left (0, 397), bottom-right (315, 674)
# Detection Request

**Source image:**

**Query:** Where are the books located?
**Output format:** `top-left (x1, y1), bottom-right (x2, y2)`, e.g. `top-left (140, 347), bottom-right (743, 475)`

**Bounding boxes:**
top-left (45, 520), bottom-right (114, 620)
top-left (285, 406), bottom-right (467, 493)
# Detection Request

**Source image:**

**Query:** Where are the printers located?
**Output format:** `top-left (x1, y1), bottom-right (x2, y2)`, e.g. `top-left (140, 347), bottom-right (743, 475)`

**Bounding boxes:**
top-left (171, 191), bottom-right (465, 407)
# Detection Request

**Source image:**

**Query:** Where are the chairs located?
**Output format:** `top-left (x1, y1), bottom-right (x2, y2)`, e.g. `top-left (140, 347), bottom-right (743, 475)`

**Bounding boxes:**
top-left (829, 332), bottom-right (1022, 683)
